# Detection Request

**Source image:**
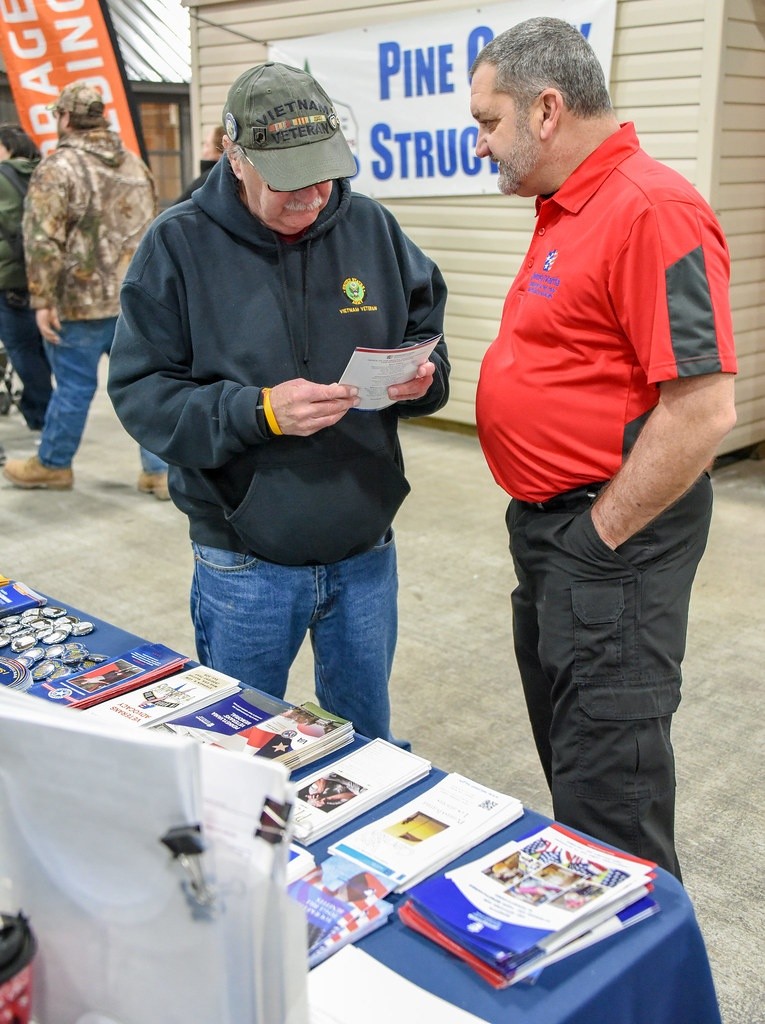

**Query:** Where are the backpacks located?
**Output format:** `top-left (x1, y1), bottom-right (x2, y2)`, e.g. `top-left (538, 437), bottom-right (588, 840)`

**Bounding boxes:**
top-left (1, 163), bottom-right (30, 262)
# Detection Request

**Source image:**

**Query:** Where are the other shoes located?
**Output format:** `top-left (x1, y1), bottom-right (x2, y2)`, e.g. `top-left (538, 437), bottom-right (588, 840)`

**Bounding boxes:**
top-left (5, 456), bottom-right (76, 491)
top-left (137, 472), bottom-right (172, 501)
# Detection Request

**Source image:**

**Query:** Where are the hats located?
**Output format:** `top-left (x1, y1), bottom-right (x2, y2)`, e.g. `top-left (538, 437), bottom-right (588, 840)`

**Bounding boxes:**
top-left (221, 63), bottom-right (357, 191)
top-left (46, 83), bottom-right (104, 117)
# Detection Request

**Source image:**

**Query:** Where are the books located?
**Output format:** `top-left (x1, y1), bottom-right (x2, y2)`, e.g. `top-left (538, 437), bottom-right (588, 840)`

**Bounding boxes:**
top-left (0, 572), bottom-right (665, 996)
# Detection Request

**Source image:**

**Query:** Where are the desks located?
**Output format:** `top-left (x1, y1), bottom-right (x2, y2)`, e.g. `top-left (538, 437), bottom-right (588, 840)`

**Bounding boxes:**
top-left (0, 573), bottom-right (724, 1024)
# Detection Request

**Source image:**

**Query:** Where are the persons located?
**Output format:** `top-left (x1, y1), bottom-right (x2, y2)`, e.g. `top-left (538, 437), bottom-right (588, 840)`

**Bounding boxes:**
top-left (463, 15), bottom-right (739, 894)
top-left (1, 78), bottom-right (180, 502)
top-left (0, 122), bottom-right (64, 431)
top-left (104, 60), bottom-right (456, 752)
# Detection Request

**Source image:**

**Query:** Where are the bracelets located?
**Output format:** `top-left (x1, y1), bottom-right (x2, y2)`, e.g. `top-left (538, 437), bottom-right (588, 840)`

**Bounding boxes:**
top-left (259, 385), bottom-right (284, 436)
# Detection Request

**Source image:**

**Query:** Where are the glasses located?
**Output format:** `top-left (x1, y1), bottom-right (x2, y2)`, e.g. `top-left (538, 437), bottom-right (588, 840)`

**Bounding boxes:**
top-left (237, 145), bottom-right (333, 191)
top-left (52, 108), bottom-right (70, 118)
